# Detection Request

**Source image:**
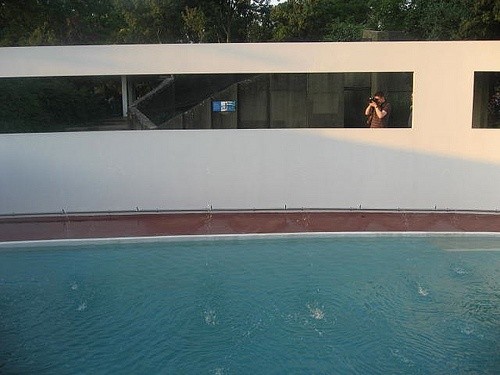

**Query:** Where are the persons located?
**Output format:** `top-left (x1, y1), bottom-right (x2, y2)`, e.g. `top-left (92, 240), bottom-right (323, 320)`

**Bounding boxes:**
top-left (364, 90), bottom-right (390, 128)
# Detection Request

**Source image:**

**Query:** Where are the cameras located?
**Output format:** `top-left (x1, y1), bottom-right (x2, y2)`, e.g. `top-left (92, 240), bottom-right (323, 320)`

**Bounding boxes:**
top-left (368, 95), bottom-right (376, 103)
